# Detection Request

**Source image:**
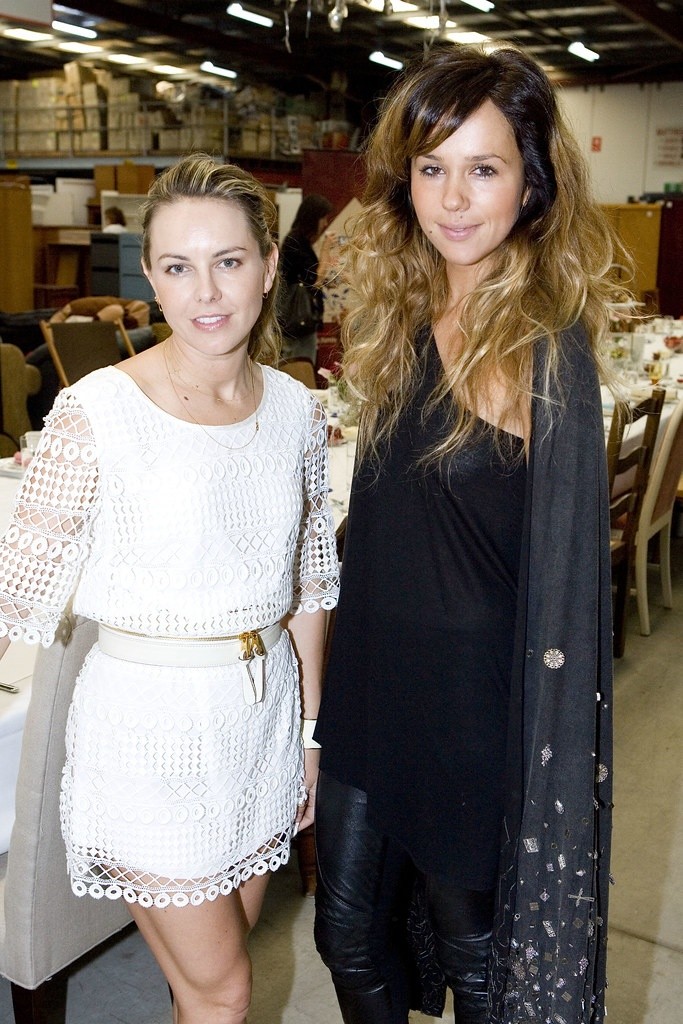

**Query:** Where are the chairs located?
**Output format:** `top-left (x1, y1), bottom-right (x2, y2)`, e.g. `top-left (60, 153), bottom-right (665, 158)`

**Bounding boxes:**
top-left (0, 613), bottom-right (174, 1024)
top-left (610, 400), bottom-right (683, 633)
top-left (39, 320), bottom-right (137, 388)
top-left (605, 388), bottom-right (668, 660)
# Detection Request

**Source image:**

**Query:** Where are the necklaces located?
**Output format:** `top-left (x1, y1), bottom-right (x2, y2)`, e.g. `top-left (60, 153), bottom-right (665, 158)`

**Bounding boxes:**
top-left (163, 337), bottom-right (259, 449)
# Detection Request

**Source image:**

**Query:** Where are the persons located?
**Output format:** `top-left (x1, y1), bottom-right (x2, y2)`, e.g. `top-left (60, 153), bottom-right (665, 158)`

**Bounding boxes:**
top-left (102, 206), bottom-right (128, 233)
top-left (305, 49), bottom-right (617, 1024)
top-left (276, 193), bottom-right (333, 344)
top-left (0, 150), bottom-right (340, 1024)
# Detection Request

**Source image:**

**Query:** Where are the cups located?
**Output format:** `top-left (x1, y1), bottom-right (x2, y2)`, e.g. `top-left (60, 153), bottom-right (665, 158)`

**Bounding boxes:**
top-left (631, 333), bottom-right (646, 362)
top-left (664, 315), bottom-right (674, 333)
top-left (20, 436), bottom-right (39, 470)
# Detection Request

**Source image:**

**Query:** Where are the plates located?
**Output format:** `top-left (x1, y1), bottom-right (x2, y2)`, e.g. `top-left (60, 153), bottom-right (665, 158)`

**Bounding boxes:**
top-left (603, 401), bottom-right (635, 417)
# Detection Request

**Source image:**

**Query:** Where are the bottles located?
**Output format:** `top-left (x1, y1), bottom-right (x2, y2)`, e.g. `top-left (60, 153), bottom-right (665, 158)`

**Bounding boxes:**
top-left (649, 353), bottom-right (662, 379)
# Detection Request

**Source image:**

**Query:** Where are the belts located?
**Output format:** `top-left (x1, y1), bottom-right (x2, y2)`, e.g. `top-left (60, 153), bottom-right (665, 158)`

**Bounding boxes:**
top-left (99, 622), bottom-right (285, 667)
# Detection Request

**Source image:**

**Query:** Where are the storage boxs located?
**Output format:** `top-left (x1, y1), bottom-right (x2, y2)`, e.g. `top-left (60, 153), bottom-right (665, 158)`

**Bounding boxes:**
top-left (1, 60), bottom-right (349, 156)
top-left (92, 164), bottom-right (154, 199)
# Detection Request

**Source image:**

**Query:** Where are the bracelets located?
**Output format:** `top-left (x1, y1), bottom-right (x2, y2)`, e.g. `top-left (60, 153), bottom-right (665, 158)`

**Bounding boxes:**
top-left (302, 718), bottom-right (322, 750)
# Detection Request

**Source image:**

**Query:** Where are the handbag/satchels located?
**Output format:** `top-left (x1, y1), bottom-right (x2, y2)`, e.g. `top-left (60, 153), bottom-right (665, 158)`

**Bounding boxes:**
top-left (277, 283), bottom-right (312, 325)
top-left (284, 286), bottom-right (325, 339)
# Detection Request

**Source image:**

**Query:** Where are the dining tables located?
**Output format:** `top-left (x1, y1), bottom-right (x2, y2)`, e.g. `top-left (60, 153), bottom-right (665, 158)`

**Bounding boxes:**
top-left (0, 318), bottom-right (683, 854)
